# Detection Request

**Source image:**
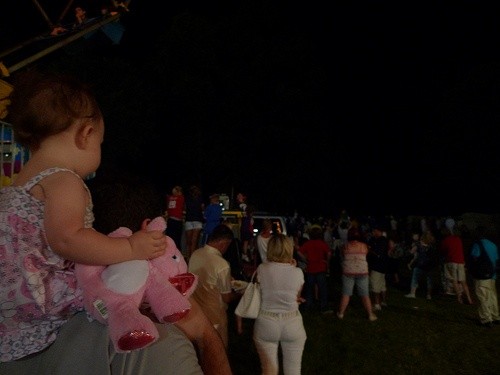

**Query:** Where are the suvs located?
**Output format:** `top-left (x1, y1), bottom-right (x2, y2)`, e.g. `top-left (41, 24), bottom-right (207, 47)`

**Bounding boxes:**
top-left (249, 209), bottom-right (288, 238)
top-left (220, 209), bottom-right (247, 240)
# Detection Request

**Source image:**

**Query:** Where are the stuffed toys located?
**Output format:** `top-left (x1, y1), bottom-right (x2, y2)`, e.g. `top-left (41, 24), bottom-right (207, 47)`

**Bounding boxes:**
top-left (75, 215), bottom-right (198, 352)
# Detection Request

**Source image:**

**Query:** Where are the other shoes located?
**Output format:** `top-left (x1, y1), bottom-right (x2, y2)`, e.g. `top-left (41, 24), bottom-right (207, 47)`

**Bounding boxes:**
top-left (426, 295), bottom-right (432, 301)
top-left (336, 312), bottom-right (344, 319)
top-left (494, 320), bottom-right (500, 325)
top-left (381, 302), bottom-right (388, 307)
top-left (404, 293), bottom-right (416, 299)
top-left (368, 316), bottom-right (378, 321)
top-left (373, 304), bottom-right (382, 311)
top-left (484, 322), bottom-right (494, 328)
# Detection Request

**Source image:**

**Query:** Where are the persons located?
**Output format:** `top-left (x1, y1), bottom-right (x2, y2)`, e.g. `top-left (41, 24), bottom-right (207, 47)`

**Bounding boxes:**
top-left (0, 184), bottom-right (500, 375)
top-left (52, 6), bottom-right (120, 40)
top-left (0, 65), bottom-right (168, 363)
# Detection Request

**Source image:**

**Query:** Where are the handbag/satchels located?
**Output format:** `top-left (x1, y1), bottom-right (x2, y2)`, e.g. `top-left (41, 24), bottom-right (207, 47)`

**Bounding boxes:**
top-left (234, 263), bottom-right (264, 319)
top-left (469, 239), bottom-right (494, 279)
